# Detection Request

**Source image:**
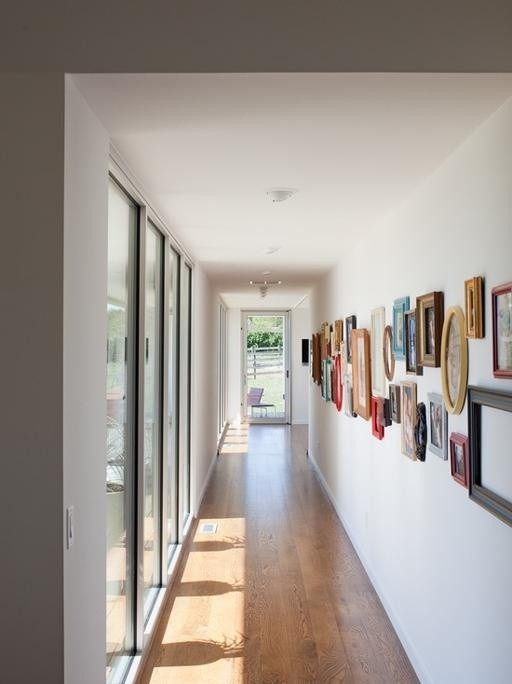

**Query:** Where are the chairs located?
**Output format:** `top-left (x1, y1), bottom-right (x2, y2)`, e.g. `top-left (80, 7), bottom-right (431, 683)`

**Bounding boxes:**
top-left (246, 386), bottom-right (265, 418)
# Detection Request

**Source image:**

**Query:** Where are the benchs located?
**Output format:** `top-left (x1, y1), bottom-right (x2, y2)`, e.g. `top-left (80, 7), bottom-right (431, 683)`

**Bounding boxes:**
top-left (251, 403), bottom-right (278, 419)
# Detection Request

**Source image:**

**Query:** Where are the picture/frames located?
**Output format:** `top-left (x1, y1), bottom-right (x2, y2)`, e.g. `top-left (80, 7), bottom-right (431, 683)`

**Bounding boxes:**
top-left (385, 291), bottom-right (447, 462)
top-left (491, 281), bottom-right (512, 380)
top-left (441, 306), bottom-right (468, 416)
top-left (464, 275), bottom-right (484, 340)
top-left (466, 385), bottom-right (511, 529)
top-left (311, 307), bottom-right (385, 440)
top-left (449, 432), bottom-right (469, 489)
